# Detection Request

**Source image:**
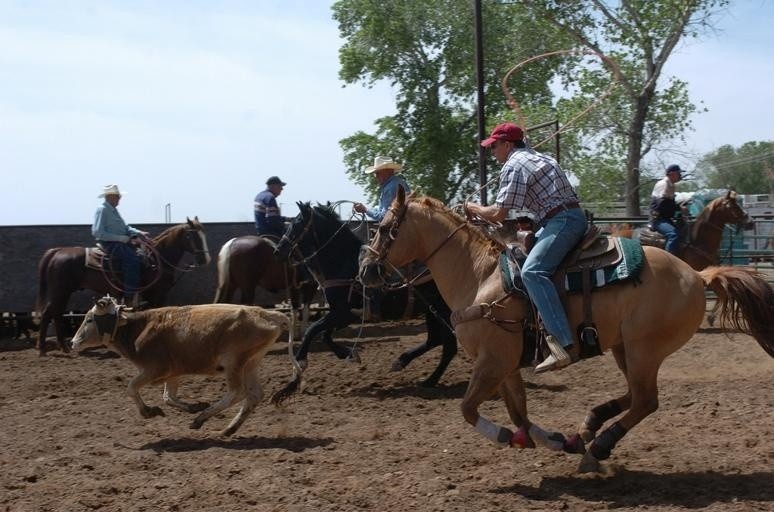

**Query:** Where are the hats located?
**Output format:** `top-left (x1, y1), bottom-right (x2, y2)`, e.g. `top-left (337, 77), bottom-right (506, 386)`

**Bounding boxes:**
top-left (481, 123), bottom-right (523, 147)
top-left (266, 176), bottom-right (286, 186)
top-left (364, 157), bottom-right (401, 173)
top-left (98, 184), bottom-right (127, 197)
top-left (666, 165), bottom-right (686, 174)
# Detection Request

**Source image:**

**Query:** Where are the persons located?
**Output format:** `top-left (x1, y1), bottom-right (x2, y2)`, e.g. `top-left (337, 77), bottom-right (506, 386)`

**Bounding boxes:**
top-left (458, 122), bottom-right (586, 370)
top-left (650, 164), bottom-right (686, 254)
top-left (89, 185), bottom-right (149, 306)
top-left (346, 156), bottom-right (409, 323)
top-left (254, 176), bottom-right (287, 237)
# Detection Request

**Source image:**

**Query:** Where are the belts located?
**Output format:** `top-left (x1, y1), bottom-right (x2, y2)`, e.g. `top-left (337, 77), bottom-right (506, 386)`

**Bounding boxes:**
top-left (546, 203), bottom-right (579, 218)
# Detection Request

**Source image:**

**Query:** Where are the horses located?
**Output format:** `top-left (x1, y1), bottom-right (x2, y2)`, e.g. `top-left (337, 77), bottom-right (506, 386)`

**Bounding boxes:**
top-left (35, 216), bottom-right (211, 358)
top-left (273, 201), bottom-right (457, 388)
top-left (608, 190), bottom-right (754, 326)
top-left (359, 183), bottom-right (773, 473)
top-left (213, 201), bottom-right (339, 322)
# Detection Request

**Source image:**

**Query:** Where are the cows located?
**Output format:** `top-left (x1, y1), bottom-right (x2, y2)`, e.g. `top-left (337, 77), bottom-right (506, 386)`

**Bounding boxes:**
top-left (68, 293), bottom-right (309, 438)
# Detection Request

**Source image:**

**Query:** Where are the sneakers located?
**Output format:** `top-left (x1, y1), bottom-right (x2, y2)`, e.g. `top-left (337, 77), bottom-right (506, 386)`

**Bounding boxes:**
top-left (127, 296), bottom-right (149, 307)
top-left (350, 308), bottom-right (372, 321)
top-left (534, 348), bottom-right (579, 372)
